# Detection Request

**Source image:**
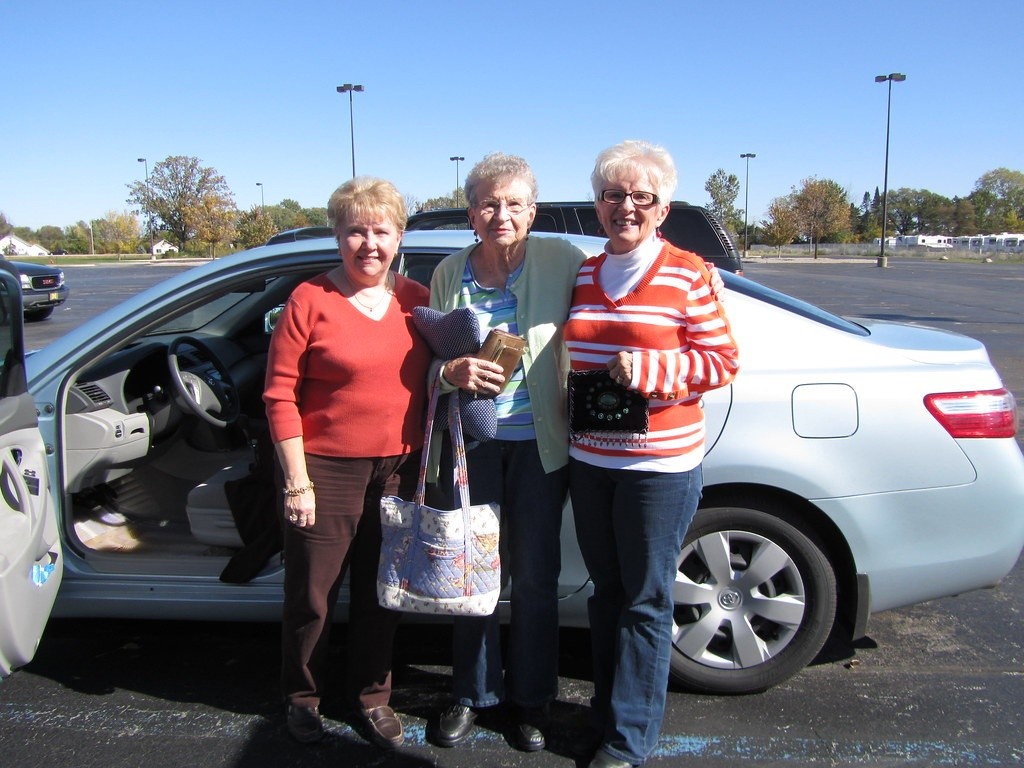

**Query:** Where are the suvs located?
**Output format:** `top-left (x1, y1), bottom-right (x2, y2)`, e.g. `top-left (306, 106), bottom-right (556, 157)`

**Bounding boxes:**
top-left (267, 200), bottom-right (743, 277)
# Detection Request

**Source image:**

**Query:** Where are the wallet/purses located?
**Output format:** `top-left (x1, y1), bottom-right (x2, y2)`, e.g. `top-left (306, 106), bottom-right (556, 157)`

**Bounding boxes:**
top-left (568, 369), bottom-right (650, 448)
top-left (473, 328), bottom-right (526, 394)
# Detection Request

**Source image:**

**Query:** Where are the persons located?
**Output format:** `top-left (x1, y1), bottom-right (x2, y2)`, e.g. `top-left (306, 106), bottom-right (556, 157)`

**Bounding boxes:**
top-left (562, 140), bottom-right (740, 768)
top-left (426, 153), bottom-right (587, 751)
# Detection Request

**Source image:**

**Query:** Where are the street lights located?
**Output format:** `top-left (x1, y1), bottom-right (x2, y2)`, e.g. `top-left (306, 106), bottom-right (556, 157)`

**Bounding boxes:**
top-left (875, 73), bottom-right (907, 256)
top-left (256, 183), bottom-right (265, 212)
top-left (740, 153), bottom-right (756, 250)
top-left (336, 83), bottom-right (364, 176)
top-left (138, 158), bottom-right (153, 245)
top-left (449, 156), bottom-right (465, 207)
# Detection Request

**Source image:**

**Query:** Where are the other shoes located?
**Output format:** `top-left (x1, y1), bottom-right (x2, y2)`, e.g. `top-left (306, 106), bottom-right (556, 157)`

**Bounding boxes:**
top-left (569, 727), bottom-right (599, 755)
top-left (586, 749), bottom-right (636, 768)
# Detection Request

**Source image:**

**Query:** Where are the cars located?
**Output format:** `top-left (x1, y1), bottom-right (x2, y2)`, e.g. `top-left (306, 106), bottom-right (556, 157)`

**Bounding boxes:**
top-left (47, 250), bottom-right (69, 255)
top-left (0, 260), bottom-right (69, 324)
top-left (0, 233), bottom-right (1024, 693)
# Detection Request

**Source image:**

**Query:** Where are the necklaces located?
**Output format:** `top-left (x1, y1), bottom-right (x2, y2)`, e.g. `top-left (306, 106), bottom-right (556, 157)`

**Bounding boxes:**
top-left (262, 177), bottom-right (431, 748)
top-left (347, 271), bottom-right (389, 312)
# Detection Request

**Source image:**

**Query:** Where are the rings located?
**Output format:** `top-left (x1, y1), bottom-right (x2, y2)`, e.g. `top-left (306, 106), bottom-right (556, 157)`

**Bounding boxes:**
top-left (289, 514), bottom-right (298, 521)
top-left (616, 375), bottom-right (623, 384)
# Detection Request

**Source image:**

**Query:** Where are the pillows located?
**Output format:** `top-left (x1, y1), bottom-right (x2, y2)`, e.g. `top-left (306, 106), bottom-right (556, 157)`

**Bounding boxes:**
top-left (411, 304), bottom-right (498, 443)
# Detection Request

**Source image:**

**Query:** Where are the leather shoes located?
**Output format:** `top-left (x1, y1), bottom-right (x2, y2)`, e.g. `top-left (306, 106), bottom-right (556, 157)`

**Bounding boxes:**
top-left (283, 691), bottom-right (324, 745)
top-left (351, 695), bottom-right (404, 749)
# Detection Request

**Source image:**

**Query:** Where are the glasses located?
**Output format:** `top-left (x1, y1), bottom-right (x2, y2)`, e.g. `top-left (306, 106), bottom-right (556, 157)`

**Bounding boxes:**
top-left (469, 198), bottom-right (532, 215)
top-left (598, 188), bottom-right (660, 207)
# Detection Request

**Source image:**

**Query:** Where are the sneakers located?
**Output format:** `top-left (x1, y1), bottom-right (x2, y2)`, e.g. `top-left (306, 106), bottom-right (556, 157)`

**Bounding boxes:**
top-left (512, 706), bottom-right (550, 751)
top-left (433, 699), bottom-right (480, 746)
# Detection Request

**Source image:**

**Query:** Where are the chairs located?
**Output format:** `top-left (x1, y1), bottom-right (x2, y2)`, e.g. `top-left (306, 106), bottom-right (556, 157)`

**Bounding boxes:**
top-left (186, 463), bottom-right (272, 549)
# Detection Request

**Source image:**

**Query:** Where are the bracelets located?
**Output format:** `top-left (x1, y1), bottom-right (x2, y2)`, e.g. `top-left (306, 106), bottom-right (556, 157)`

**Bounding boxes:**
top-left (283, 482), bottom-right (314, 496)
top-left (439, 359), bottom-right (459, 391)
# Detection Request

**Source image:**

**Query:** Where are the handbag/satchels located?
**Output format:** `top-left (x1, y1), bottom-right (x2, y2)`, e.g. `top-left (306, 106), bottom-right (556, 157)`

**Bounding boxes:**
top-left (219, 463), bottom-right (284, 583)
top-left (375, 358), bottom-right (502, 618)
